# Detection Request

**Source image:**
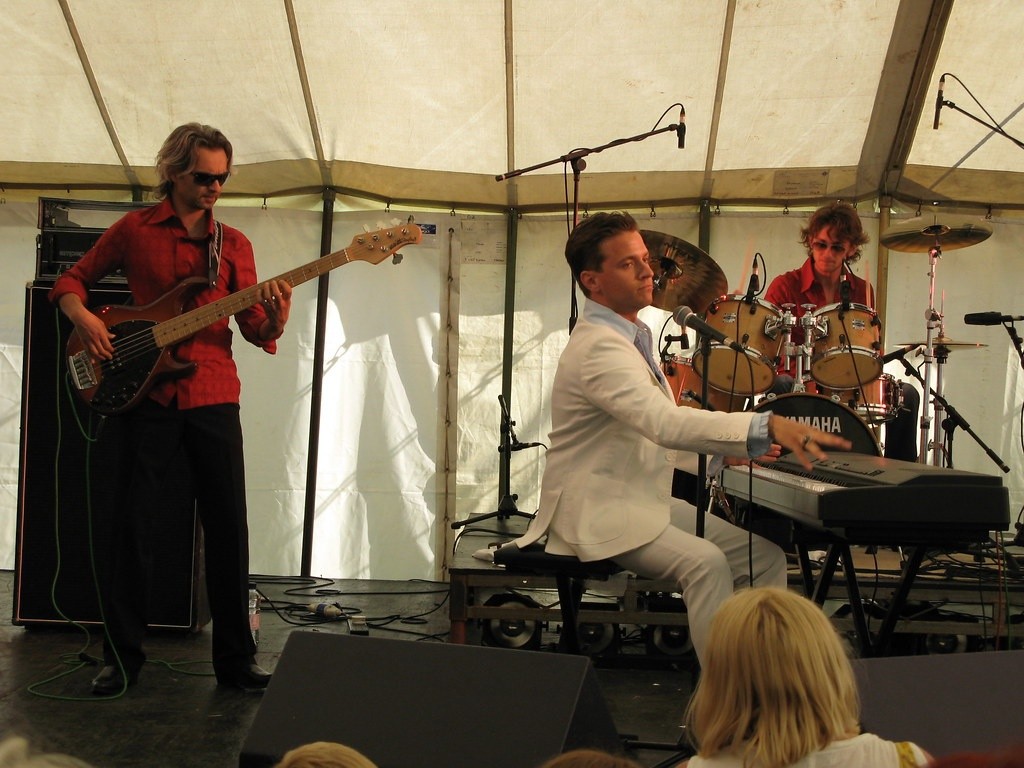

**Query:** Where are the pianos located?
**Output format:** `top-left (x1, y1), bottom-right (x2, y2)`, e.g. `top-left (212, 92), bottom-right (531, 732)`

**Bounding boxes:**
top-left (672, 451), bottom-right (1013, 657)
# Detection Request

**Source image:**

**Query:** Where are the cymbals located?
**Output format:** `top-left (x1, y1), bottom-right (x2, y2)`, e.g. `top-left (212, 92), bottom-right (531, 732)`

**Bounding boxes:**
top-left (639, 230), bottom-right (728, 315)
top-left (879, 215), bottom-right (993, 252)
top-left (895, 332), bottom-right (989, 352)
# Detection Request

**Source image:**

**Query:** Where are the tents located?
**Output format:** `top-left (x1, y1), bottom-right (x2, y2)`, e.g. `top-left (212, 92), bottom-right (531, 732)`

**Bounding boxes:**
top-left (0, 0), bottom-right (1024, 581)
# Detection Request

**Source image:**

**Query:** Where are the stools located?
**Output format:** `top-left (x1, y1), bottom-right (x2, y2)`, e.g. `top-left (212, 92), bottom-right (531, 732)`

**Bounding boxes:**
top-left (448, 511), bottom-right (688, 652)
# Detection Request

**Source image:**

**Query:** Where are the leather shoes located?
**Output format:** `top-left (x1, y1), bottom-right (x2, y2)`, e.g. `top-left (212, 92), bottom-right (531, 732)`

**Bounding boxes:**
top-left (215, 664), bottom-right (272, 688)
top-left (92, 665), bottom-right (137, 695)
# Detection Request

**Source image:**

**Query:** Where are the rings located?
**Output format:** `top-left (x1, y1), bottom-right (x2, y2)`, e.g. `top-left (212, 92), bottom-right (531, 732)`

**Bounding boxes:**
top-left (801, 435), bottom-right (814, 452)
top-left (269, 301), bottom-right (274, 306)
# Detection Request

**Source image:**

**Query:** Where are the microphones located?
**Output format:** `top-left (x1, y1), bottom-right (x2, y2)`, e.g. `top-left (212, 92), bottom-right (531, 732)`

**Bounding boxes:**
top-left (965, 312), bottom-right (1024, 326)
top-left (753, 258), bottom-right (760, 291)
top-left (672, 305), bottom-right (745, 353)
top-left (678, 108), bottom-right (686, 149)
top-left (883, 343), bottom-right (920, 365)
top-left (839, 265), bottom-right (846, 294)
top-left (499, 442), bottom-right (543, 453)
top-left (933, 76), bottom-right (946, 129)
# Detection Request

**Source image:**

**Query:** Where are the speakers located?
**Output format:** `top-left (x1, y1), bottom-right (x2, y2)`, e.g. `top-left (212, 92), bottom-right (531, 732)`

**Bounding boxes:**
top-left (12, 282), bottom-right (210, 636)
top-left (239, 630), bottom-right (628, 768)
top-left (850, 649), bottom-right (1024, 757)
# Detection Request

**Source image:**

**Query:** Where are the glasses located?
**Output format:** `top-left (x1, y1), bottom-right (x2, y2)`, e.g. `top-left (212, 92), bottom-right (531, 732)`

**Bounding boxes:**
top-left (190, 172), bottom-right (231, 186)
top-left (813, 238), bottom-right (849, 252)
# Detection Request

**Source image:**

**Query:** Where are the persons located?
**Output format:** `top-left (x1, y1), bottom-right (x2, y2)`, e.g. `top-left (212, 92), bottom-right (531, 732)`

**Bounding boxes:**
top-left (764, 204), bottom-right (920, 463)
top-left (675, 586), bottom-right (934, 768)
top-left (536, 749), bottom-right (638, 768)
top-left (276, 742), bottom-right (377, 768)
top-left (0, 736), bottom-right (92, 768)
top-left (48, 122), bottom-right (292, 692)
top-left (471, 211), bottom-right (852, 670)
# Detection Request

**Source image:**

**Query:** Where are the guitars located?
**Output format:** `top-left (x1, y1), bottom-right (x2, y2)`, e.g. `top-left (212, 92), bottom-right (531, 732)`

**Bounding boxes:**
top-left (64, 215), bottom-right (423, 417)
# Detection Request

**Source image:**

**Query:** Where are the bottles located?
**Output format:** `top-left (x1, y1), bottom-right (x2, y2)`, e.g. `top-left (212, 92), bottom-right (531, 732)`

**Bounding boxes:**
top-left (247, 582), bottom-right (260, 646)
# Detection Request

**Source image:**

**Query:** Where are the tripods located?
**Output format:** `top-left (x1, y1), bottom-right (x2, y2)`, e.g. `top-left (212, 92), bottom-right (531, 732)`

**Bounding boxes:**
top-left (450, 395), bottom-right (537, 530)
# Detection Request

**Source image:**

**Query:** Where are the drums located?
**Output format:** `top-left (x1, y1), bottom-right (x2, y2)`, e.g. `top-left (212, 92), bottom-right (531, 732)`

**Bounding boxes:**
top-left (717, 392), bottom-right (882, 564)
top-left (691, 294), bottom-right (785, 396)
top-left (801, 372), bottom-right (903, 421)
top-left (658, 355), bottom-right (746, 451)
top-left (810, 302), bottom-right (884, 391)
